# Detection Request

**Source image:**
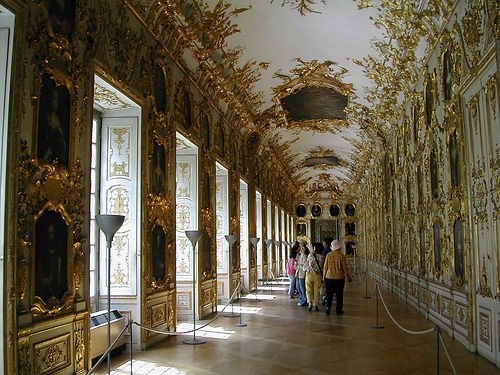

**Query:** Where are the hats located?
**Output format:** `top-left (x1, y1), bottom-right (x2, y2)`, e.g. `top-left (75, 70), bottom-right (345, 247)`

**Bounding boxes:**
top-left (329, 240), bottom-right (342, 250)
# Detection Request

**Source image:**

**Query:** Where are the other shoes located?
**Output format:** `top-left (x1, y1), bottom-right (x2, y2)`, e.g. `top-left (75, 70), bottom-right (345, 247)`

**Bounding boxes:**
top-left (308, 303), bottom-right (312, 311)
top-left (289, 296), bottom-right (292, 299)
top-left (315, 306), bottom-right (318, 311)
top-left (296, 303), bottom-right (301, 305)
top-left (336, 311), bottom-right (344, 315)
top-left (321, 296), bottom-right (327, 306)
top-left (325, 310), bottom-right (330, 315)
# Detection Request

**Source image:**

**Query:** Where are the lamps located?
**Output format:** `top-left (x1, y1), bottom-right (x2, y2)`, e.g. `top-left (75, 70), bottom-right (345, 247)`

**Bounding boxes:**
top-left (95, 214), bottom-right (126, 375)
top-left (249, 237), bottom-right (288, 302)
top-left (183, 231), bottom-right (207, 344)
top-left (222, 235), bottom-right (241, 317)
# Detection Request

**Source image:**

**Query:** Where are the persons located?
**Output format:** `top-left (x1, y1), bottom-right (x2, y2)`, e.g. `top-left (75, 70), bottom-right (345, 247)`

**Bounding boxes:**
top-left (287, 236), bottom-right (353, 316)
